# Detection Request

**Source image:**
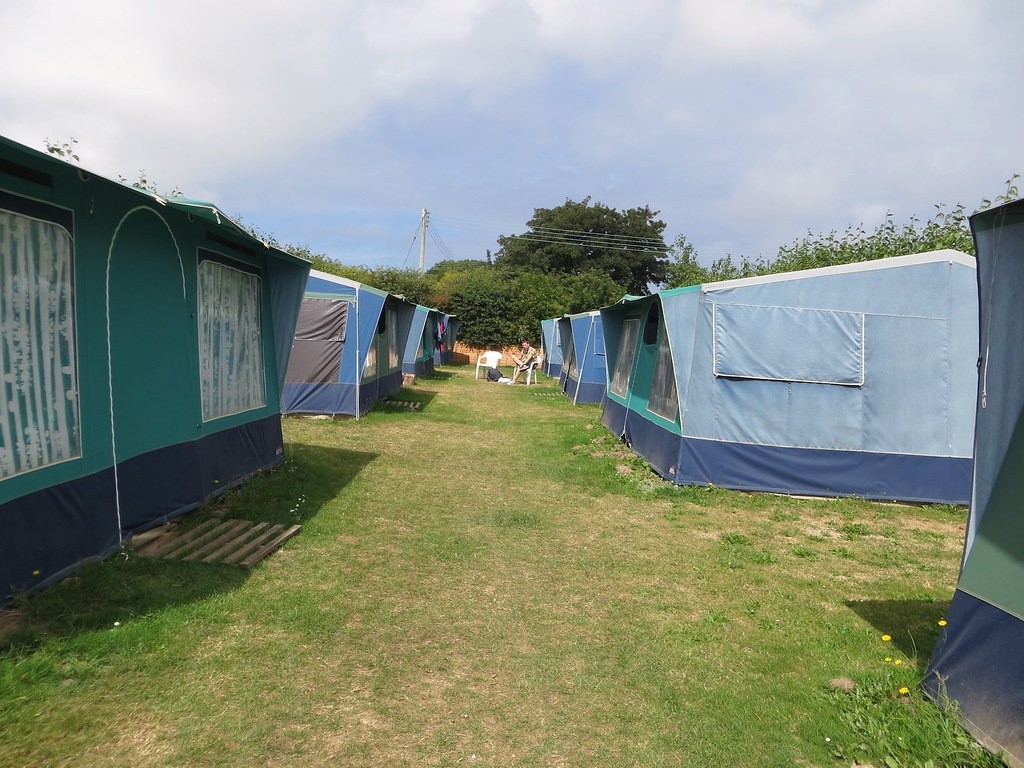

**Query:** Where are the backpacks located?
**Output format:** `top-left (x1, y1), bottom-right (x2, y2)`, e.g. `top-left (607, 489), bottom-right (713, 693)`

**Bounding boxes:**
top-left (486, 367), bottom-right (504, 382)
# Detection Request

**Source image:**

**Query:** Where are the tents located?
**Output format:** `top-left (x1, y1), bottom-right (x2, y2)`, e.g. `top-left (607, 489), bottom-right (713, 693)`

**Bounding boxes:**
top-left (280, 268), bottom-right (418, 421)
top-left (919, 199), bottom-right (1024, 768)
top-left (597, 249), bottom-right (979, 506)
top-left (402, 304), bottom-right (461, 376)
top-left (0, 139), bottom-right (315, 616)
top-left (540, 311), bottom-right (606, 405)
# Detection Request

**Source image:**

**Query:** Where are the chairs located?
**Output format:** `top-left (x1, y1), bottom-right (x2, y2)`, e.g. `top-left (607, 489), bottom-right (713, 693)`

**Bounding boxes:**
top-left (476, 352), bottom-right (503, 378)
top-left (513, 356), bottom-right (541, 384)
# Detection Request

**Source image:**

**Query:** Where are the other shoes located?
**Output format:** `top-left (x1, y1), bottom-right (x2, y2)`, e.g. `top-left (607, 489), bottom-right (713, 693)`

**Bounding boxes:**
top-left (519, 365), bottom-right (530, 372)
top-left (507, 381), bottom-right (517, 385)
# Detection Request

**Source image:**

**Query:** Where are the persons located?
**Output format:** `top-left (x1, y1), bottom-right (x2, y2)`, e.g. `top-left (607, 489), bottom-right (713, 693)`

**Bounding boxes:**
top-left (506, 341), bottom-right (539, 385)
